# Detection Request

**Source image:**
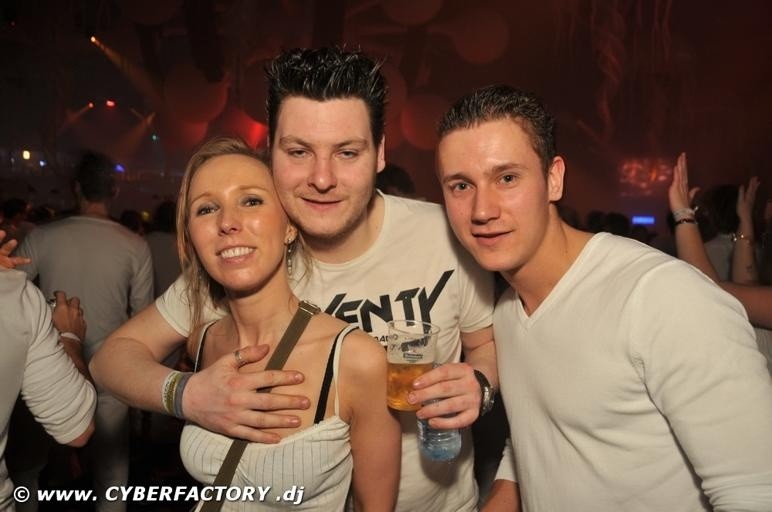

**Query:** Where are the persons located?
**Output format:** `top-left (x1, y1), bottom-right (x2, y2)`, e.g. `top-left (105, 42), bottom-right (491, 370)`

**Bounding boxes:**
top-left (88, 40), bottom-right (501, 510)
top-left (169, 131), bottom-right (406, 510)
top-left (1, 150), bottom-right (772, 511)
top-left (434, 82), bottom-right (772, 509)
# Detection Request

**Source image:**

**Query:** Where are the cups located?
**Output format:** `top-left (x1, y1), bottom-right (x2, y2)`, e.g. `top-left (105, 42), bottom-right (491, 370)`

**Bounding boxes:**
top-left (387, 319), bottom-right (462, 462)
